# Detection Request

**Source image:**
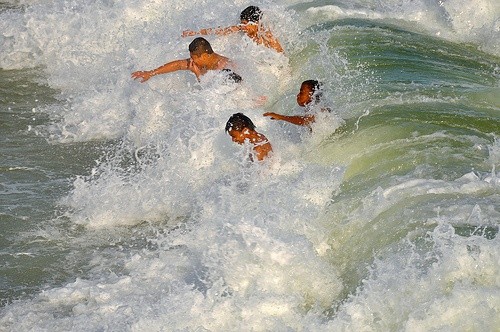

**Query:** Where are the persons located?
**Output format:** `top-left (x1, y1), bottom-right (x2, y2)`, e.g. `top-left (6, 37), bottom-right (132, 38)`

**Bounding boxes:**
top-left (262, 81), bottom-right (330, 134)
top-left (180, 4), bottom-right (283, 57)
top-left (130, 37), bottom-right (239, 87)
top-left (225, 114), bottom-right (272, 164)
